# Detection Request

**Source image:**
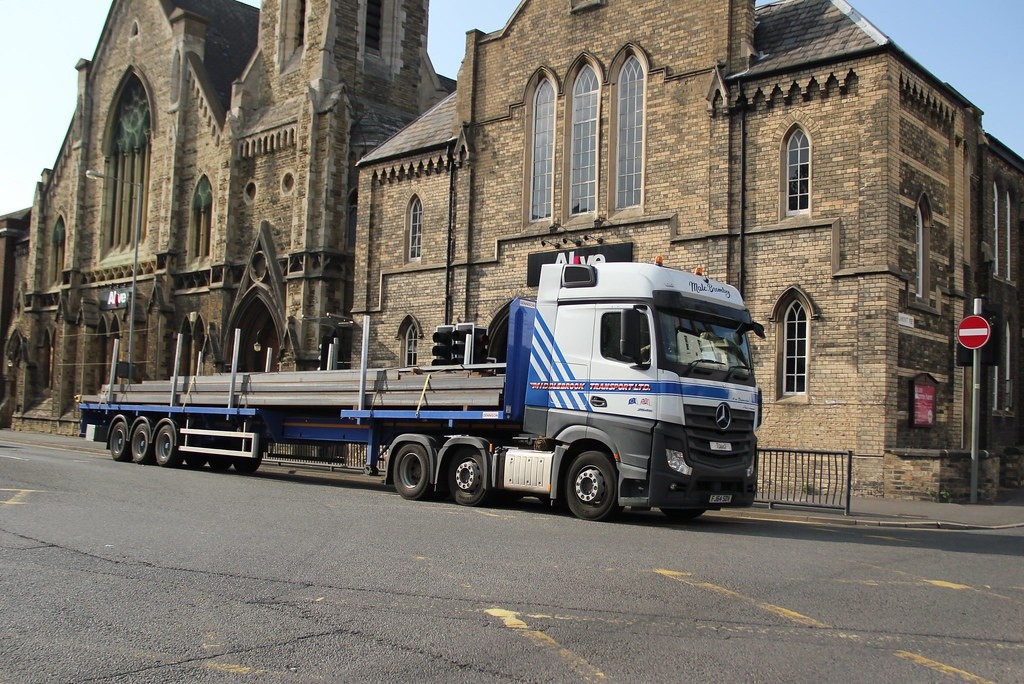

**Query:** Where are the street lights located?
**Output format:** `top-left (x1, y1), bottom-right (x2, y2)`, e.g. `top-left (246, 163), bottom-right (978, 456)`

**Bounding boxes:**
top-left (87, 170), bottom-right (144, 384)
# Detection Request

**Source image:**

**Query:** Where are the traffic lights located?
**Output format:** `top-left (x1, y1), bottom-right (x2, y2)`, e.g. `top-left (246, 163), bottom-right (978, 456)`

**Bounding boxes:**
top-left (474, 326), bottom-right (490, 366)
top-left (431, 325), bottom-right (452, 366)
top-left (452, 323), bottom-right (475, 368)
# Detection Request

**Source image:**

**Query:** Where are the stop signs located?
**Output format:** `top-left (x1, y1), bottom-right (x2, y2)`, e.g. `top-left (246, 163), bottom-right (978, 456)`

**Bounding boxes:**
top-left (956, 315), bottom-right (991, 349)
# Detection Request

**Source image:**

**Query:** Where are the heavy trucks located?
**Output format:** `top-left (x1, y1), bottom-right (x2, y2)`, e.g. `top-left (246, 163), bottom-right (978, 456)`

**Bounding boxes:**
top-left (73, 256), bottom-right (765, 521)
